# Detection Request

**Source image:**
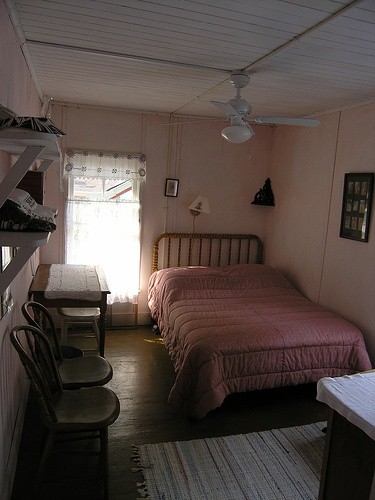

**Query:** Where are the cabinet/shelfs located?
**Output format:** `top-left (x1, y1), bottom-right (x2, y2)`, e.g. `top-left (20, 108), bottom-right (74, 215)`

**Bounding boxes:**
top-left (0, 106), bottom-right (67, 298)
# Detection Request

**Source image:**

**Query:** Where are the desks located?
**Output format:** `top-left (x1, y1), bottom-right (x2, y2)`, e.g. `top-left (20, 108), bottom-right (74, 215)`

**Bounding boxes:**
top-left (314, 369), bottom-right (375, 500)
top-left (28, 263), bottom-right (111, 358)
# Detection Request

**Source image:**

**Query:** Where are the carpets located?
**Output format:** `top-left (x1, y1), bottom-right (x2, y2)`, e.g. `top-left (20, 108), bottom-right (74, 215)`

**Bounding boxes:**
top-left (130, 421), bottom-right (328, 500)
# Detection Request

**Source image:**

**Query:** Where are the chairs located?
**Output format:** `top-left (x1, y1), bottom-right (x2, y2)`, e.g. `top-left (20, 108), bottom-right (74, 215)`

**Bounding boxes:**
top-left (10, 325), bottom-right (120, 500)
top-left (21, 301), bottom-right (113, 456)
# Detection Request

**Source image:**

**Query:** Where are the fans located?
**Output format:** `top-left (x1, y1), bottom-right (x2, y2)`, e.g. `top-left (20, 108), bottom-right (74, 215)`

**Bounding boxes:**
top-left (159, 70), bottom-right (320, 136)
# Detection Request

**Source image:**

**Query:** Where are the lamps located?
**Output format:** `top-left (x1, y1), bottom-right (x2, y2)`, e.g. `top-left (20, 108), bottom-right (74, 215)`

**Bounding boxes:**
top-left (220, 126), bottom-right (251, 143)
top-left (186, 195), bottom-right (210, 234)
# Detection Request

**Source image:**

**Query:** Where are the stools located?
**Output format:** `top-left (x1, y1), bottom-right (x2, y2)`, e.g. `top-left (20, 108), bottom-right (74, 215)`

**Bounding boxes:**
top-left (57, 308), bottom-right (101, 351)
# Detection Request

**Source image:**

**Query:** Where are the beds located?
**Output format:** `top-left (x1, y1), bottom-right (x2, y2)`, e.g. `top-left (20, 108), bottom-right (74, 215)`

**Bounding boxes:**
top-left (147, 233), bottom-right (373, 421)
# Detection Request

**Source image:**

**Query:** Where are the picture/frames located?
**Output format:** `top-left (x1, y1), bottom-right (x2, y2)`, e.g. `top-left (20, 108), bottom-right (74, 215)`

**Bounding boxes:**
top-left (164, 178), bottom-right (179, 197)
top-left (339, 172), bottom-right (375, 243)
